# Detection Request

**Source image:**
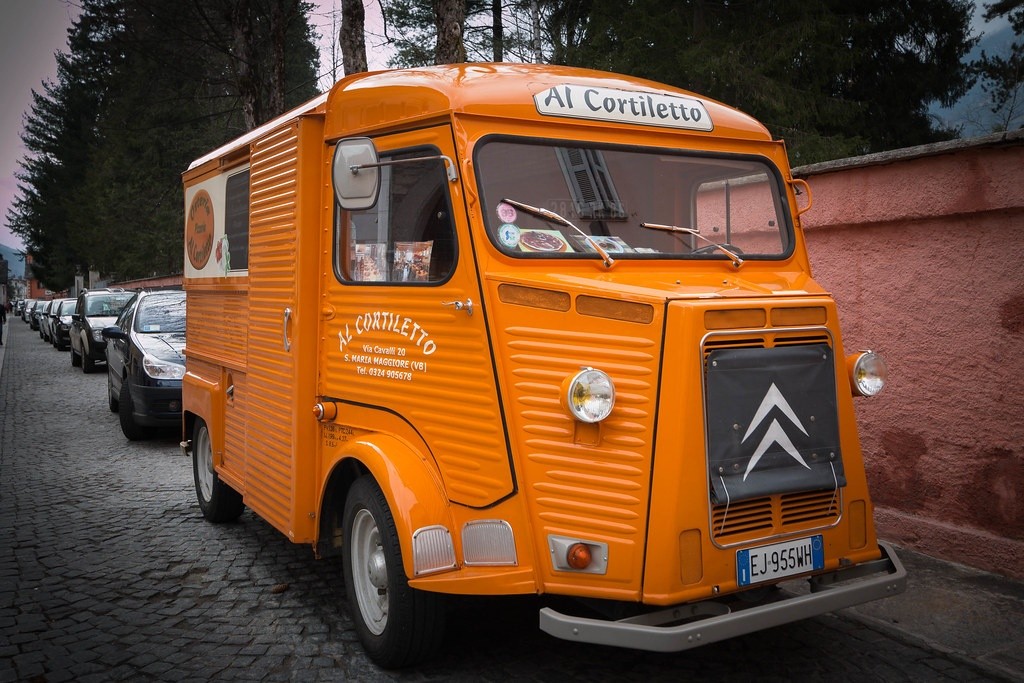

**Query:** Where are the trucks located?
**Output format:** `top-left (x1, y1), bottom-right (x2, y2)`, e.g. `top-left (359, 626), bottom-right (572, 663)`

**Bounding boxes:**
top-left (180, 63), bottom-right (910, 670)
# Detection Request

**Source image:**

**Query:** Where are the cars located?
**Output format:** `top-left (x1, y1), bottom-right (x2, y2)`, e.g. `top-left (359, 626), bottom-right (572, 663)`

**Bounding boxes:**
top-left (14, 298), bottom-right (110, 352)
top-left (102, 289), bottom-right (190, 444)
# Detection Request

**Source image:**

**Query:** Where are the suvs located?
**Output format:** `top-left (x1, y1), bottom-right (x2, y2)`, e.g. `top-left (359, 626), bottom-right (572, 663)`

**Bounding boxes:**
top-left (70, 288), bottom-right (139, 373)
